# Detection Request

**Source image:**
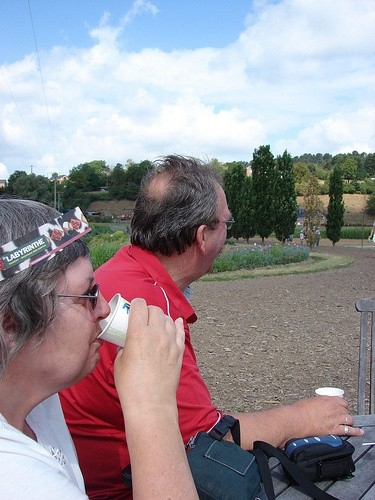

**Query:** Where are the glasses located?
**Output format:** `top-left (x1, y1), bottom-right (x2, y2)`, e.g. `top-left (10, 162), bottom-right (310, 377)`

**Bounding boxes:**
top-left (49, 282), bottom-right (98, 310)
top-left (209, 218), bottom-right (236, 230)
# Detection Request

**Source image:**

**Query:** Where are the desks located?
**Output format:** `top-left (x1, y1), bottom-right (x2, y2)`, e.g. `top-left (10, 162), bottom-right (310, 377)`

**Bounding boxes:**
top-left (256, 413), bottom-right (375, 500)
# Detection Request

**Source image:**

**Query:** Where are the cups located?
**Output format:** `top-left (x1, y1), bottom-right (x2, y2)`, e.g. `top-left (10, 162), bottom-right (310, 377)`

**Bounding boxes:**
top-left (96, 293), bottom-right (132, 347)
top-left (315, 387), bottom-right (345, 398)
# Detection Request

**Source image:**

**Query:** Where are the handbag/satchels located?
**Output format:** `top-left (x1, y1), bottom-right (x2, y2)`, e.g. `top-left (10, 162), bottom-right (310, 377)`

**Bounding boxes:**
top-left (185, 431), bottom-right (265, 500)
top-left (281, 433), bottom-right (355, 482)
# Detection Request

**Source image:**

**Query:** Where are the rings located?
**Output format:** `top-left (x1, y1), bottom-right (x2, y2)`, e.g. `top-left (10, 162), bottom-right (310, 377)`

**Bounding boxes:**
top-left (344, 425), bottom-right (349, 434)
top-left (344, 416), bottom-right (347, 424)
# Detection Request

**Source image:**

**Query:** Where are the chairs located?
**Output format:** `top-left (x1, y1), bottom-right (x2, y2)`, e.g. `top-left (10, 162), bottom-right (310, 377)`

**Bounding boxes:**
top-left (355, 295), bottom-right (375, 416)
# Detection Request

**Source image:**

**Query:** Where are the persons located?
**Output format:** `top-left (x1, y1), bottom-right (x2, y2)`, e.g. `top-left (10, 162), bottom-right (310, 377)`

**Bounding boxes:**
top-left (300, 230), bottom-right (304, 244)
top-left (0, 198), bottom-right (200, 500)
top-left (316, 227), bottom-right (320, 246)
top-left (56, 154), bottom-right (366, 500)
